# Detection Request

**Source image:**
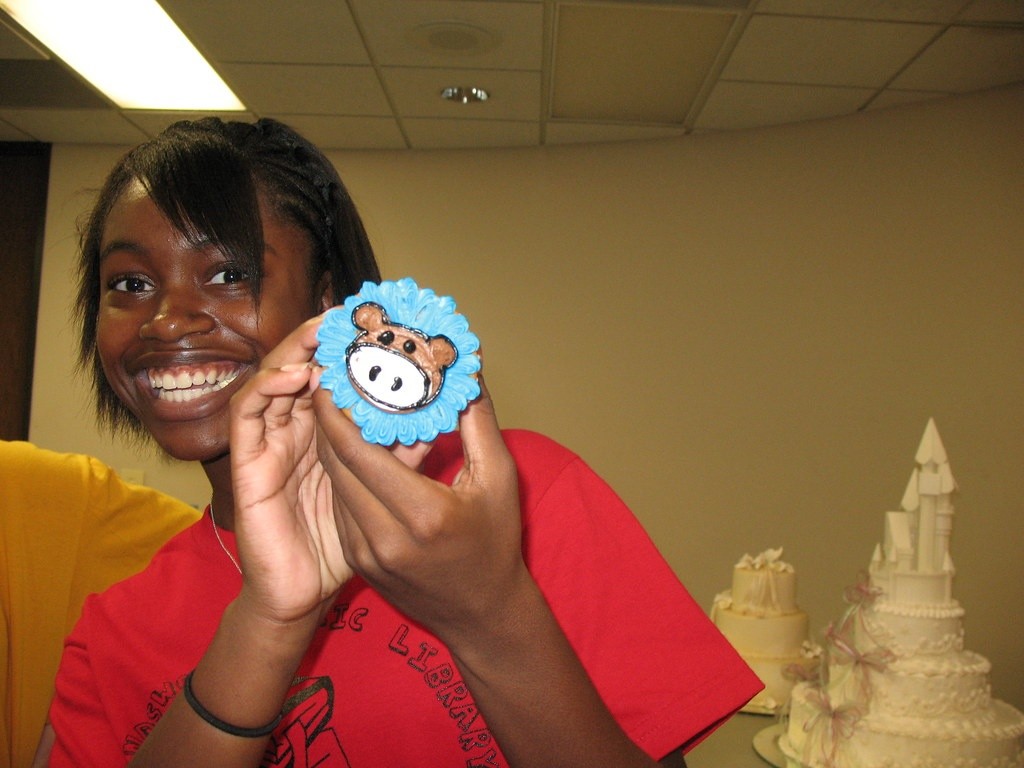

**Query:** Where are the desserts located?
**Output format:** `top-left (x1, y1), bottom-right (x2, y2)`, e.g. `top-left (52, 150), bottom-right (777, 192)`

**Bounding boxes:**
top-left (312, 277), bottom-right (484, 447)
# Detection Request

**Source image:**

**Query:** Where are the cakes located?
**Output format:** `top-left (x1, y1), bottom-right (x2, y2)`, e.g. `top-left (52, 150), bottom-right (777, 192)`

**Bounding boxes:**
top-left (709, 546), bottom-right (824, 711)
top-left (776, 418), bottom-right (1024, 767)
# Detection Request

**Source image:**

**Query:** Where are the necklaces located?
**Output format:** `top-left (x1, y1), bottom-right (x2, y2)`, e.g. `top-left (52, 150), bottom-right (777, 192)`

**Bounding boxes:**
top-left (208, 498), bottom-right (244, 578)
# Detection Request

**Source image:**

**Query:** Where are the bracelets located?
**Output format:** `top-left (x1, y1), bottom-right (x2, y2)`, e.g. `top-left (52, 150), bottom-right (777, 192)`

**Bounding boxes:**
top-left (184, 671), bottom-right (282, 737)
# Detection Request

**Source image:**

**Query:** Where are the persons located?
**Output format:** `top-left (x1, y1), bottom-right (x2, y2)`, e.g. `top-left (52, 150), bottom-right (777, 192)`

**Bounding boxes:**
top-left (0, 438), bottom-right (205, 768)
top-left (33, 114), bottom-right (767, 768)
